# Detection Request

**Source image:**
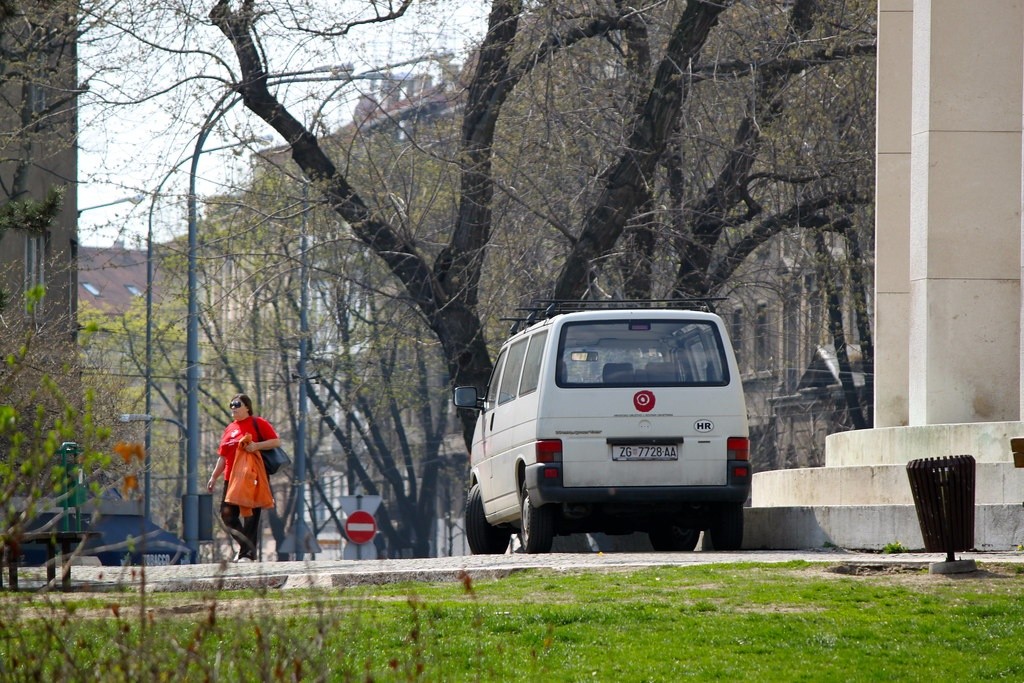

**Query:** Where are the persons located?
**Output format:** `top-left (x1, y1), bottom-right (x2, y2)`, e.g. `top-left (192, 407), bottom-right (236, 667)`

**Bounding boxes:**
top-left (206, 393), bottom-right (283, 563)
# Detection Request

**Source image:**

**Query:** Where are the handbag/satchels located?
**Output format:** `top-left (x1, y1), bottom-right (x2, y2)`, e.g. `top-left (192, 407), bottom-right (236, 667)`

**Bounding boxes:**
top-left (254, 417), bottom-right (291, 475)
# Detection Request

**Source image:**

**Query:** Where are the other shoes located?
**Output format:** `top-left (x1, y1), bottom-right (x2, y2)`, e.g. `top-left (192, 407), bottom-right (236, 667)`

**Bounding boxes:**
top-left (233, 552), bottom-right (240, 563)
top-left (238, 557), bottom-right (253, 563)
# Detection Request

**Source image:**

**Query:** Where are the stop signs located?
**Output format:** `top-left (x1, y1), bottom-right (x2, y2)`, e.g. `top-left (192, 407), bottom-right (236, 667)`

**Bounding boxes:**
top-left (345, 510), bottom-right (377, 544)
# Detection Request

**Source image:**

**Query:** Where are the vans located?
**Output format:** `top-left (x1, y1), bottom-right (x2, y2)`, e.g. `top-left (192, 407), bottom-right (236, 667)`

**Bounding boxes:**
top-left (453, 301), bottom-right (754, 553)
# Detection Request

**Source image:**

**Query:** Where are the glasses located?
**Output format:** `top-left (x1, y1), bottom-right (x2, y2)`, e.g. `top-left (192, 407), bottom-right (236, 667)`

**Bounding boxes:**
top-left (229, 401), bottom-right (246, 409)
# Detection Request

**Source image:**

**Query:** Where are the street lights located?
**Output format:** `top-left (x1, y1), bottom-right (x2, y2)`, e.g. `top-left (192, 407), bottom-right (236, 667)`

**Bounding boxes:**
top-left (119, 63), bottom-right (354, 566)
top-left (140, 136), bottom-right (273, 516)
